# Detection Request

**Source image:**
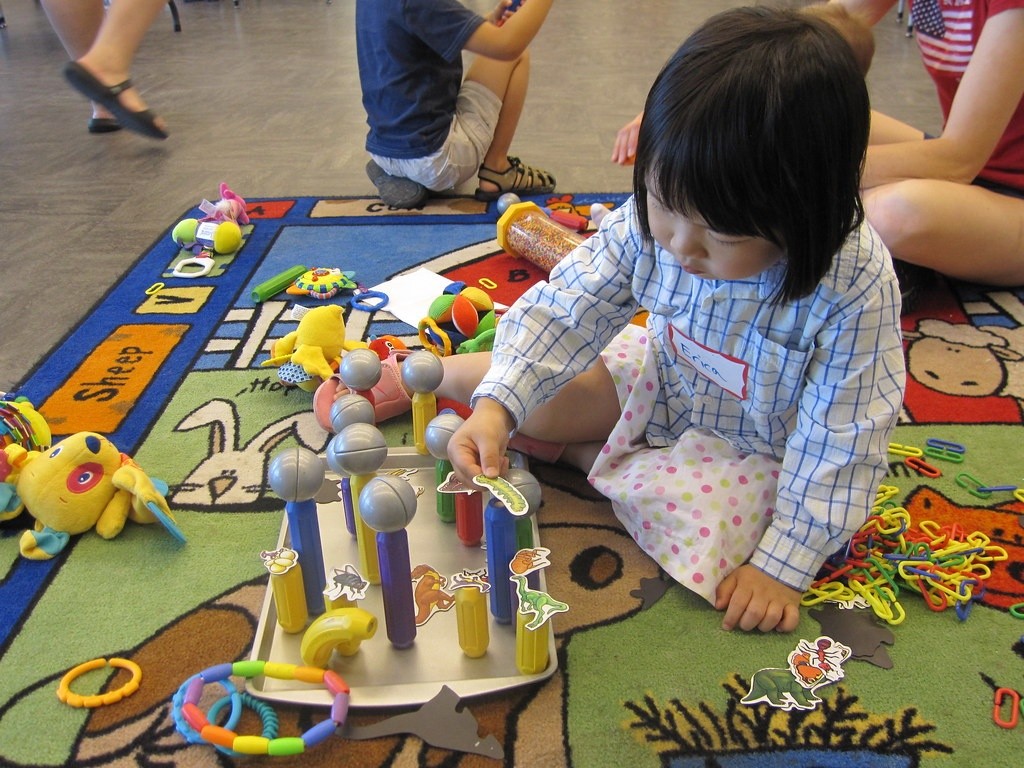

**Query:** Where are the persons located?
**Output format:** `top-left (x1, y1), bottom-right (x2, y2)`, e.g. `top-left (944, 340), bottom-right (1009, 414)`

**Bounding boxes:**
top-left (258, 305), bottom-right (377, 396)
top-left (353, 0), bottom-right (557, 205)
top-left (42, 1), bottom-right (176, 140)
top-left (315, 8), bottom-right (907, 635)
top-left (0, 431), bottom-right (187, 567)
top-left (609, 0), bottom-right (1023, 286)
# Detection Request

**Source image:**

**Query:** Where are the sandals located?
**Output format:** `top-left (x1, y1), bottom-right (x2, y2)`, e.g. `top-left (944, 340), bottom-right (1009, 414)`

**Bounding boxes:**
top-left (364, 158), bottom-right (427, 210)
top-left (475, 154), bottom-right (558, 201)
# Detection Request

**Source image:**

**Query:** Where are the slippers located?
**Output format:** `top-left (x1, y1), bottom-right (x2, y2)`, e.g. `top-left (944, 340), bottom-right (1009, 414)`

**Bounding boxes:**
top-left (62, 58), bottom-right (171, 145)
top-left (310, 347), bottom-right (420, 432)
top-left (83, 114), bottom-right (121, 132)
